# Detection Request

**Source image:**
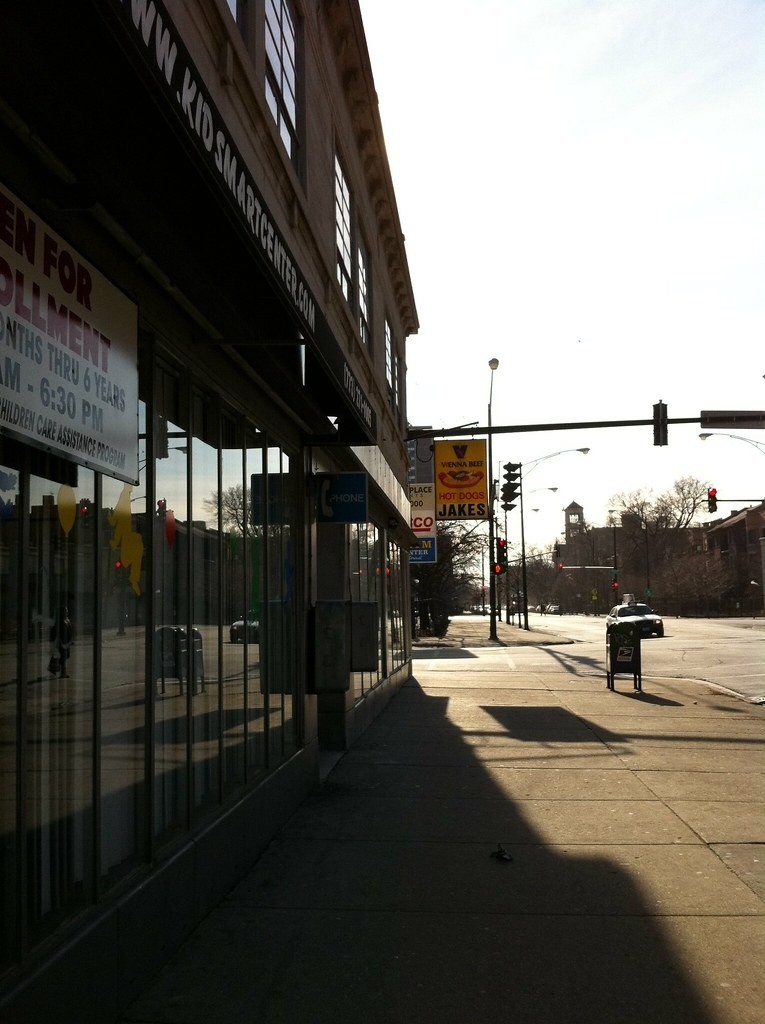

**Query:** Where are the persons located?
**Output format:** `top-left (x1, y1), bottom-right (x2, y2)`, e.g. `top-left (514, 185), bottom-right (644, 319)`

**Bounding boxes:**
top-left (51, 605), bottom-right (72, 678)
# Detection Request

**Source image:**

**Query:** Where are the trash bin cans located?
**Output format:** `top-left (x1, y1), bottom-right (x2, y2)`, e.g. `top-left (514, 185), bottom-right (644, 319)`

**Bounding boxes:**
top-left (605, 622), bottom-right (642, 690)
top-left (151, 626), bottom-right (190, 696)
top-left (185, 627), bottom-right (206, 695)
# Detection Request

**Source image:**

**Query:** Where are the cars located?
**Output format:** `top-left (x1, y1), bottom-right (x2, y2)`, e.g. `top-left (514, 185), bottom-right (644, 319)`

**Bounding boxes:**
top-left (607, 593), bottom-right (665, 640)
top-left (528, 604), bottom-right (560, 615)
top-left (227, 608), bottom-right (259, 643)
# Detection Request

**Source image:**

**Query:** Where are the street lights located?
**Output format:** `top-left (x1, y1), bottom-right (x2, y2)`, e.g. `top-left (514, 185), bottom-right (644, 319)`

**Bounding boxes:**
top-left (487, 359), bottom-right (499, 641)
top-left (519, 448), bottom-right (591, 629)
top-left (498, 509), bottom-right (542, 622)
top-left (504, 485), bottom-right (559, 623)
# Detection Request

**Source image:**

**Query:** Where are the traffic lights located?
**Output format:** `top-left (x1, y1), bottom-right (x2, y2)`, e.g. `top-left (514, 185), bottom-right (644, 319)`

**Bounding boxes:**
top-left (497, 539), bottom-right (506, 564)
top-left (158, 499), bottom-right (165, 521)
top-left (357, 569), bottom-right (363, 575)
top-left (495, 563), bottom-right (507, 575)
top-left (705, 488), bottom-right (718, 513)
top-left (115, 561), bottom-right (122, 569)
top-left (501, 462), bottom-right (522, 511)
top-left (481, 585), bottom-right (486, 591)
top-left (81, 506), bottom-right (90, 528)
top-left (612, 578), bottom-right (618, 590)
top-left (558, 563), bottom-right (563, 570)
top-left (374, 559), bottom-right (392, 577)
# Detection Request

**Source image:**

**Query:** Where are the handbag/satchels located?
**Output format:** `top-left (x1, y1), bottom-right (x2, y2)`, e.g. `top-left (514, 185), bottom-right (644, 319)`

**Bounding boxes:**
top-left (47, 655), bottom-right (60, 675)
